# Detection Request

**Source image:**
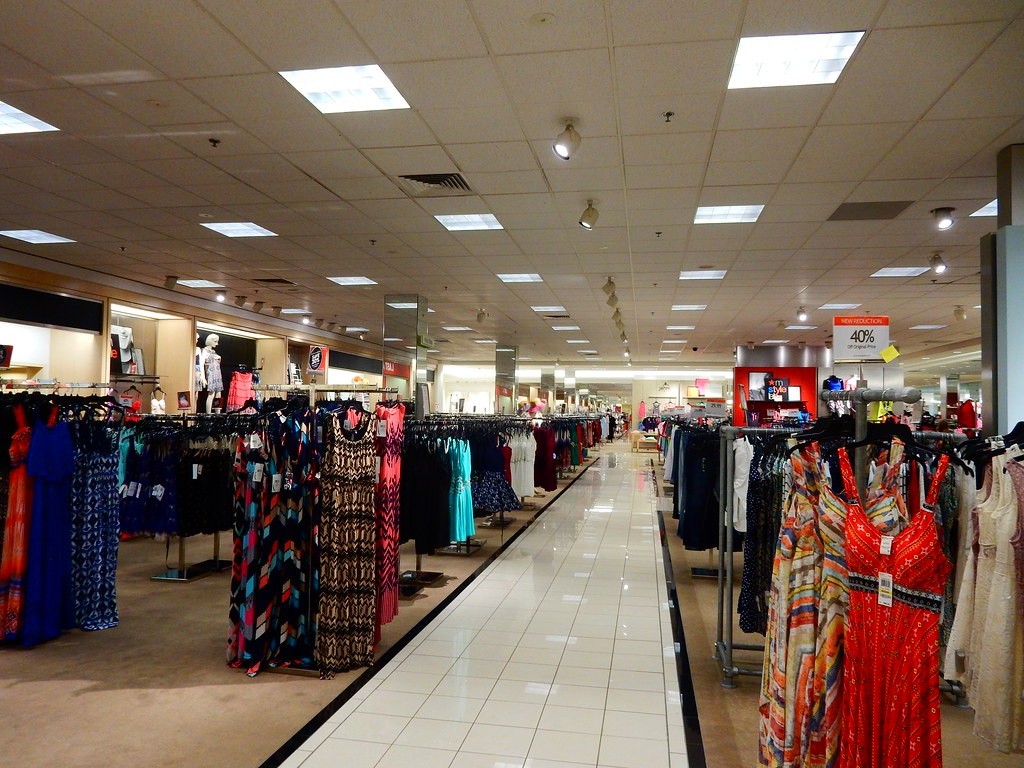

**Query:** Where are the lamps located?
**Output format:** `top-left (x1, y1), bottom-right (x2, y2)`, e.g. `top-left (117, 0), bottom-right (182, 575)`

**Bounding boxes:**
top-left (601, 276), bottom-right (632, 367)
top-left (748, 342), bottom-right (754, 351)
top-left (551, 119), bottom-right (582, 162)
top-left (797, 307), bottom-right (808, 322)
top-left (252, 301), bottom-right (265, 313)
top-left (932, 209), bottom-right (956, 232)
top-left (338, 326), bottom-right (347, 335)
top-left (272, 306), bottom-right (281, 318)
top-left (775, 321), bottom-right (786, 335)
top-left (475, 310), bottom-right (490, 323)
top-left (234, 296), bottom-right (246, 308)
top-left (576, 200), bottom-right (600, 230)
top-left (556, 360), bottom-right (560, 365)
top-left (825, 342), bottom-right (833, 349)
top-left (360, 331), bottom-right (368, 340)
top-left (164, 276), bottom-right (177, 289)
top-left (798, 343), bottom-right (806, 350)
top-left (313, 318), bottom-right (324, 328)
top-left (326, 322), bottom-right (337, 331)
top-left (928, 254), bottom-right (948, 276)
top-left (953, 306), bottom-right (967, 322)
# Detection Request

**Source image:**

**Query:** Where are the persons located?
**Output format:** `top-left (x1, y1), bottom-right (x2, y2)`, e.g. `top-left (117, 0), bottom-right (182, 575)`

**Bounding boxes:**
top-left (823, 374), bottom-right (860, 409)
top-left (195, 332), bottom-right (223, 414)
top-left (111, 325), bottom-right (134, 373)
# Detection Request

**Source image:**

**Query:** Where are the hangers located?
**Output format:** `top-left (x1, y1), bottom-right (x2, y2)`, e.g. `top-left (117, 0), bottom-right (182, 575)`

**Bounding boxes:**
top-left (639, 388), bottom-right (1024, 479)
top-left (0, 375), bottom-right (612, 443)
